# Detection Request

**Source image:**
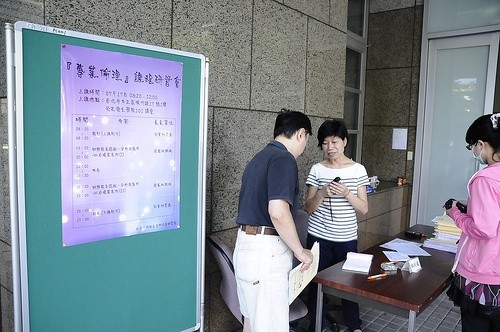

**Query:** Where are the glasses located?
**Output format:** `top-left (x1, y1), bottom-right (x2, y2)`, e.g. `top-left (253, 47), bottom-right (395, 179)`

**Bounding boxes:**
top-left (466, 142), bottom-right (478, 150)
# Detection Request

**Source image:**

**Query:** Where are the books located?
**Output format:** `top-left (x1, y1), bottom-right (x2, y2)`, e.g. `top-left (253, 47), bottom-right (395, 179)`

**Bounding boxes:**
top-left (342, 252), bottom-right (373, 273)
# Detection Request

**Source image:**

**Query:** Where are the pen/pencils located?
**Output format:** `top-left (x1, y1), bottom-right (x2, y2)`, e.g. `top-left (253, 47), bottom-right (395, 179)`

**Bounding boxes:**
top-left (382, 261), bottom-right (398, 264)
top-left (367, 273), bottom-right (388, 279)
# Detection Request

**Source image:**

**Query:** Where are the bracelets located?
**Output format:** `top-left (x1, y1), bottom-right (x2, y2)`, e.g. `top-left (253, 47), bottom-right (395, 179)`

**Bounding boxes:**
top-left (345, 191), bottom-right (350, 196)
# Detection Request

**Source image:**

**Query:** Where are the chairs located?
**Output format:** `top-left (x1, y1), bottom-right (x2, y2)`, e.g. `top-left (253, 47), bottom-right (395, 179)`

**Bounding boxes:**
top-left (206, 235), bottom-right (310, 332)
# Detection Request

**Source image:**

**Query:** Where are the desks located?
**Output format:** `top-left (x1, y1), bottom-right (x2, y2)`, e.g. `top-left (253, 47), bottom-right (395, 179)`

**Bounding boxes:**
top-left (311, 224), bottom-right (457, 332)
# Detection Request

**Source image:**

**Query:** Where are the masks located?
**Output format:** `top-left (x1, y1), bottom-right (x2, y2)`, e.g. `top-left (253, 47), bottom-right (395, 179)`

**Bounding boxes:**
top-left (472, 143), bottom-right (486, 165)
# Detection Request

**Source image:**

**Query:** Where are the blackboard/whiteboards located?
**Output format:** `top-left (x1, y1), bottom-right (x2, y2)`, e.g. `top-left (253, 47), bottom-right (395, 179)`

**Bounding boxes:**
top-left (4, 20), bottom-right (211, 330)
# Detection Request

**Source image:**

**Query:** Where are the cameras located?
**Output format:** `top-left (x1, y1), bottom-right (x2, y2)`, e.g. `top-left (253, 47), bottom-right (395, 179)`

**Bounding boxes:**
top-left (445, 198), bottom-right (466, 214)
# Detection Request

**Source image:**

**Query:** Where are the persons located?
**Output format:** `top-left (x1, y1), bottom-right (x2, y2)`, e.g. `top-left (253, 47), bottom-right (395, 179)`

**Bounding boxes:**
top-left (305, 119), bottom-right (370, 332)
top-left (446, 114), bottom-right (500, 332)
top-left (232, 108), bottom-right (313, 332)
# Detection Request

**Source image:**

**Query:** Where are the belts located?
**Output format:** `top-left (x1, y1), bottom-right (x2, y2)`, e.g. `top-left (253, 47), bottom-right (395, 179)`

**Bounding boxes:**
top-left (241, 225), bottom-right (280, 236)
